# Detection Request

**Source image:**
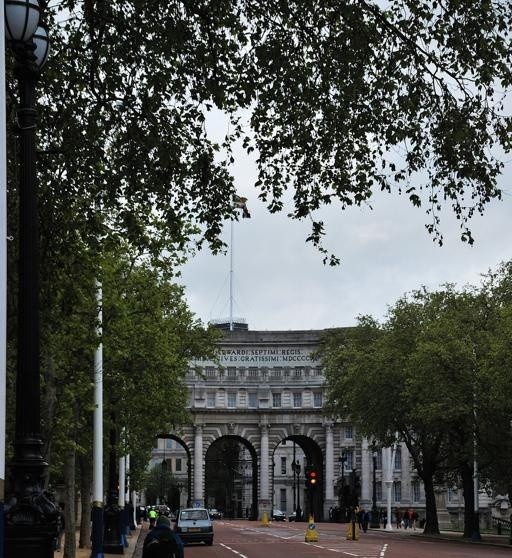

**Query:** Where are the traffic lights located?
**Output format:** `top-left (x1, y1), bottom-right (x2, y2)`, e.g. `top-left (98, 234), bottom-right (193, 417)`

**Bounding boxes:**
top-left (307, 466), bottom-right (318, 487)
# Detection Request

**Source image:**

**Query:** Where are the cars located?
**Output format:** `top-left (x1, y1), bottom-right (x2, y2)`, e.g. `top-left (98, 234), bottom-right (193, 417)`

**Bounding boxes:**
top-left (170, 507), bottom-right (216, 545)
top-left (208, 508), bottom-right (221, 519)
top-left (273, 509), bottom-right (304, 522)
top-left (141, 504), bottom-right (172, 517)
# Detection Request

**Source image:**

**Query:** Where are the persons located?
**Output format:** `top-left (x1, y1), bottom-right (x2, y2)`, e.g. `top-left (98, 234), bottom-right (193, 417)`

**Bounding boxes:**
top-left (53, 497), bottom-right (184, 558)
top-left (207, 503), bottom-right (250, 521)
top-left (328, 505), bottom-right (419, 534)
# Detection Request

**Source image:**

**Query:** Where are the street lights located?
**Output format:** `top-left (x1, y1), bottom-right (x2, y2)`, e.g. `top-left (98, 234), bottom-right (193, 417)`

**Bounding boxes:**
top-left (368, 438), bottom-right (381, 527)
top-left (93, 304), bottom-right (129, 556)
top-left (6, 1), bottom-right (66, 558)
top-left (338, 453), bottom-right (349, 524)
top-left (294, 460), bottom-right (303, 522)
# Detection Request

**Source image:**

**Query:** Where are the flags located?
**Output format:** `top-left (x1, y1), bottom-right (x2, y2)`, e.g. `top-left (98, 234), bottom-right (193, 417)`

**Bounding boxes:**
top-left (233, 193), bottom-right (248, 210)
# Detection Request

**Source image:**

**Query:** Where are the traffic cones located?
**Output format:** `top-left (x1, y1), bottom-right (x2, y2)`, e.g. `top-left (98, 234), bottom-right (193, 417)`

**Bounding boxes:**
top-left (303, 513), bottom-right (320, 541)
top-left (260, 510), bottom-right (269, 525)
